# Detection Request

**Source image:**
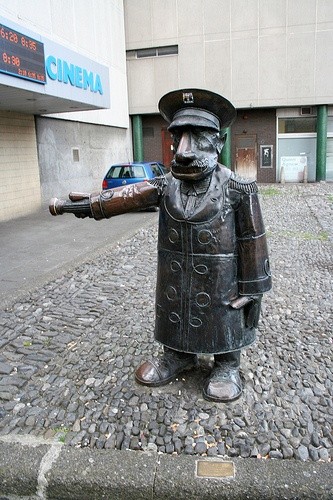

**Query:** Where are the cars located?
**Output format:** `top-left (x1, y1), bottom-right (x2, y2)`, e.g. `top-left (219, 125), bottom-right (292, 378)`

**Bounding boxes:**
top-left (101, 162), bottom-right (173, 213)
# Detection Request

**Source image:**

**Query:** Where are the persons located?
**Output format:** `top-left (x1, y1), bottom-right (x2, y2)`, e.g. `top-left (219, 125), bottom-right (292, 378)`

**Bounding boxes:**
top-left (68, 111), bottom-right (271, 402)
